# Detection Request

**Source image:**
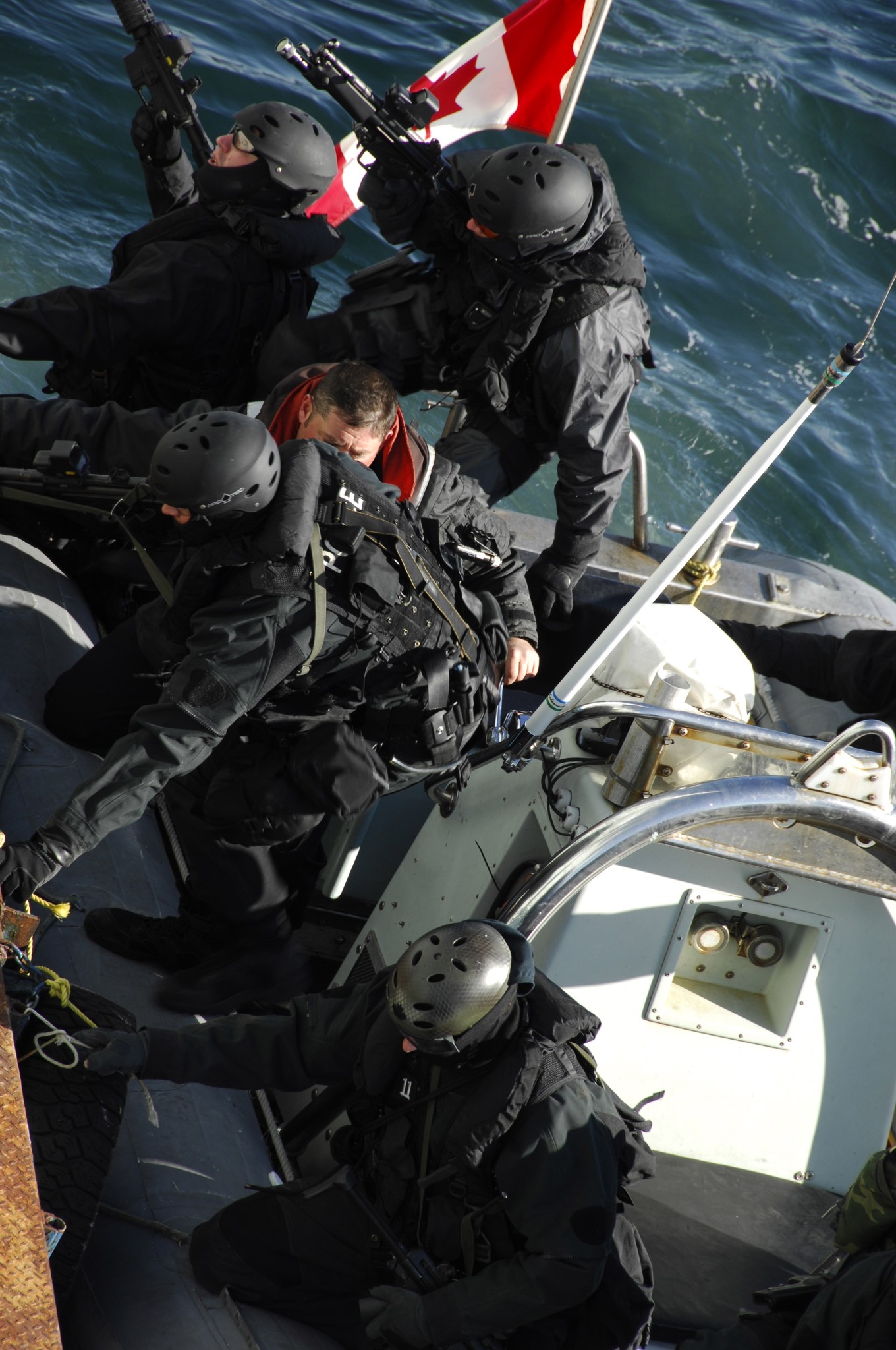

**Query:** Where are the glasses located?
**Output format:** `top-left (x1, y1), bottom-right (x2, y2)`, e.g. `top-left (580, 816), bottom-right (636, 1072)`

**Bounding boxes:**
top-left (228, 123), bottom-right (263, 159)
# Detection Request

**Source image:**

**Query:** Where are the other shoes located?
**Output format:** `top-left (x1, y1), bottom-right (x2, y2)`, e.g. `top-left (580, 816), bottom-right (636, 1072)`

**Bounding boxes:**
top-left (87, 904), bottom-right (305, 1012)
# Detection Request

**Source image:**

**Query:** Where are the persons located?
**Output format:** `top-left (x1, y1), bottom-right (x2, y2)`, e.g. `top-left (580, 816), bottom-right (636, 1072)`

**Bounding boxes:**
top-left (71, 914), bottom-right (652, 1350)
top-left (0, 394), bottom-right (506, 906)
top-left (254, 140), bottom-right (650, 622)
top-left (254, 356), bottom-right (543, 689)
top-left (0, 99), bottom-right (343, 407)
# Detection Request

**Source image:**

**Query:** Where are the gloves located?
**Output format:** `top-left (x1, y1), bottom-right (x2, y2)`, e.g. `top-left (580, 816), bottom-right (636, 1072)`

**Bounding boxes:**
top-left (365, 1284), bottom-right (435, 1350)
top-left (526, 549), bottom-right (587, 619)
top-left (68, 1023), bottom-right (146, 1077)
top-left (0, 831), bottom-right (61, 907)
top-left (130, 103), bottom-right (181, 160)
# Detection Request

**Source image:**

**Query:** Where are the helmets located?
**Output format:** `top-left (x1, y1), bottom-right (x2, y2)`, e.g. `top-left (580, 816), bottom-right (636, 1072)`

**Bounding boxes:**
top-left (462, 141), bottom-right (593, 247)
top-left (387, 922), bottom-right (512, 1051)
top-left (145, 407), bottom-right (283, 514)
top-left (232, 101), bottom-right (339, 218)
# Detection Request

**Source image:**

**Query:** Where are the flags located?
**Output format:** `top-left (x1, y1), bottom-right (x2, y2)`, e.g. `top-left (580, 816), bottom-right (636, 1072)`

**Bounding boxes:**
top-left (305, 0), bottom-right (612, 227)
